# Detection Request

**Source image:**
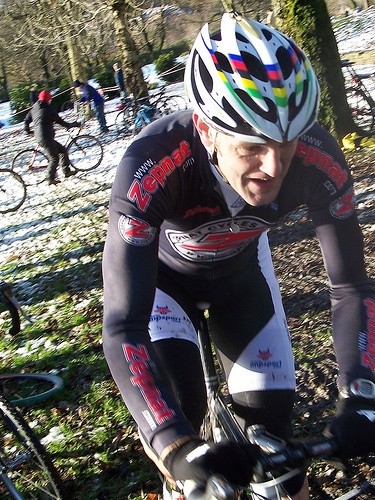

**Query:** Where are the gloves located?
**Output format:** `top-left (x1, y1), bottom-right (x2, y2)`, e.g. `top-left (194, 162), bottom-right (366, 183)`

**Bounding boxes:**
top-left (323, 368), bottom-right (375, 449)
top-left (163, 436), bottom-right (261, 500)
top-left (73, 121), bottom-right (81, 127)
top-left (28, 131), bottom-right (33, 134)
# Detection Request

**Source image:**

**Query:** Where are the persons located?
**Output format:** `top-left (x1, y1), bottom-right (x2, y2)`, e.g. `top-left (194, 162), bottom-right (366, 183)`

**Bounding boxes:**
top-left (74, 80), bottom-right (109, 136)
top-left (23, 91), bottom-right (82, 185)
top-left (99, 13), bottom-right (375, 500)
top-left (113, 62), bottom-right (130, 119)
top-left (28, 86), bottom-right (38, 106)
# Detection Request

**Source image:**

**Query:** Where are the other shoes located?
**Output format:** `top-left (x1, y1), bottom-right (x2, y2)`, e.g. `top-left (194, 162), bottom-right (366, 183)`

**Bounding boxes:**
top-left (98, 128), bottom-right (109, 135)
top-left (49, 177), bottom-right (61, 185)
top-left (65, 171), bottom-right (76, 177)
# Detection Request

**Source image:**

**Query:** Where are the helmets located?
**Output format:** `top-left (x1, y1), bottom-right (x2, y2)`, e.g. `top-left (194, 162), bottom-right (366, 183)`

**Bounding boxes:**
top-left (184, 11), bottom-right (320, 148)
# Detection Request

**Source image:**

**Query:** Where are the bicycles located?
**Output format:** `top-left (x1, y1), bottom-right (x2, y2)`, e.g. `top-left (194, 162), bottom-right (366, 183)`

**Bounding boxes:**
top-left (188, 299), bottom-right (375, 500)
top-left (114, 84), bottom-right (187, 141)
top-left (0, 279), bottom-right (69, 500)
top-left (11, 117), bottom-right (104, 187)
top-left (340, 58), bottom-right (375, 132)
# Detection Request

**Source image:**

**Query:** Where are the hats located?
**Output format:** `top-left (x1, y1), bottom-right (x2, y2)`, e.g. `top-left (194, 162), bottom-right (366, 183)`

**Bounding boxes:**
top-left (74, 80), bottom-right (81, 88)
top-left (38, 90), bottom-right (52, 101)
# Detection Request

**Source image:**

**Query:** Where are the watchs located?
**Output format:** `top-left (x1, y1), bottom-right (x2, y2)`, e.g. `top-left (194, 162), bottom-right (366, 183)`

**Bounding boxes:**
top-left (338, 379), bottom-right (375, 399)
